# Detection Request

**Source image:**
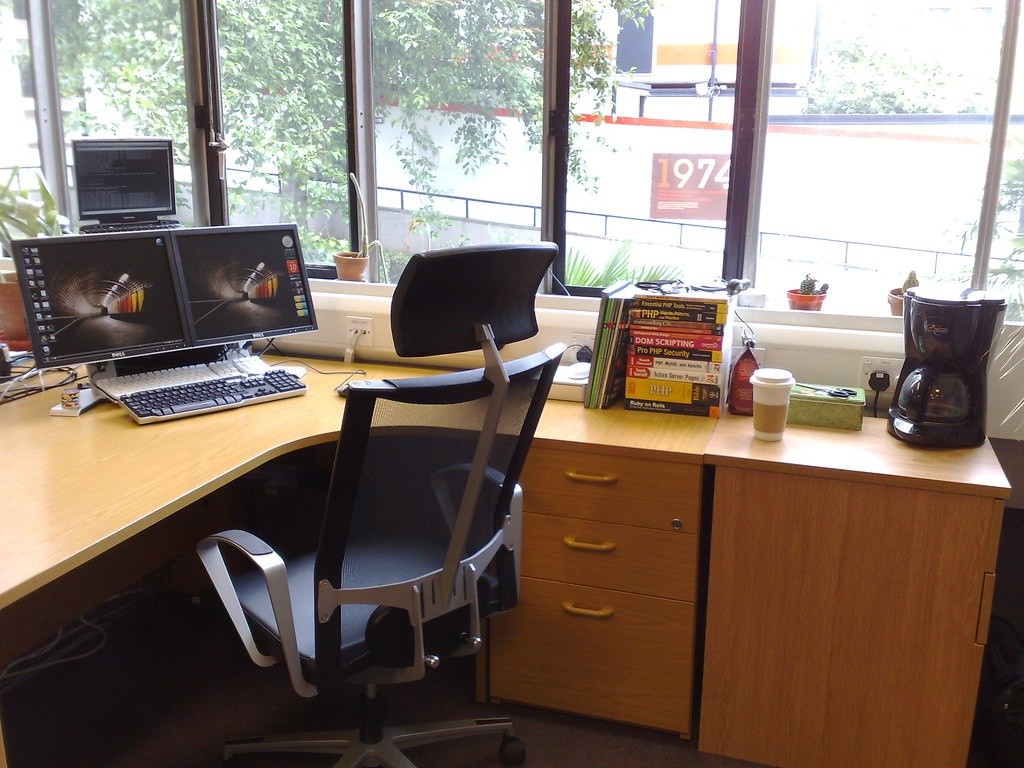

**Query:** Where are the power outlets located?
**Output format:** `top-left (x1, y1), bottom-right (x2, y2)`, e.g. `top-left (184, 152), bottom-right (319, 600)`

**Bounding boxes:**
top-left (861, 356), bottom-right (907, 394)
top-left (345, 316), bottom-right (373, 346)
top-left (571, 333), bottom-right (596, 364)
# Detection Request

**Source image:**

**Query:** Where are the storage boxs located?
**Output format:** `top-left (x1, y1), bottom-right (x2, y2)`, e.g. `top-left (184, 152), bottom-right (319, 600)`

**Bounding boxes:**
top-left (787, 383), bottom-right (866, 431)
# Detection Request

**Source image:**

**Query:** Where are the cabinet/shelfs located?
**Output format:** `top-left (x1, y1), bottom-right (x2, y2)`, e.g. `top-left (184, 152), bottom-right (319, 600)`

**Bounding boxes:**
top-left (698, 403), bottom-right (1014, 768)
top-left (474, 434), bottom-right (703, 741)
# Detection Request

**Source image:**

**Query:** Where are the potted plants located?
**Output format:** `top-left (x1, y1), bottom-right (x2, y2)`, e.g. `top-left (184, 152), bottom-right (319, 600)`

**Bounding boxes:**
top-left (887, 270), bottom-right (919, 316)
top-left (333, 173), bottom-right (391, 284)
top-left (0, 165), bottom-right (63, 340)
top-left (787, 273), bottom-right (829, 311)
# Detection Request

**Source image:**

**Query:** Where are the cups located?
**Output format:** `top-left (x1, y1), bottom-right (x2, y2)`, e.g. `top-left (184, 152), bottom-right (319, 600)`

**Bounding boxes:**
top-left (60, 388), bottom-right (80, 408)
top-left (748, 368), bottom-right (796, 441)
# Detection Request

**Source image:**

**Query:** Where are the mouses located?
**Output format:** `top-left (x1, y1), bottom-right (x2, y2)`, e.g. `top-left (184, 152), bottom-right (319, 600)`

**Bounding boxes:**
top-left (338, 384), bottom-right (350, 397)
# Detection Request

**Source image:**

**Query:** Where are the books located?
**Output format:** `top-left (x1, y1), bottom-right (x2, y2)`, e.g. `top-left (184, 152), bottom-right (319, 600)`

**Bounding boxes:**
top-left (582, 279), bottom-right (737, 418)
top-left (548, 367), bottom-right (592, 403)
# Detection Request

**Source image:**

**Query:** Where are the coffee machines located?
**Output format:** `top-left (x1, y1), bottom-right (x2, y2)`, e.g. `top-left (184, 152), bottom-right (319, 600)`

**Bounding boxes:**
top-left (886, 286), bottom-right (1008, 449)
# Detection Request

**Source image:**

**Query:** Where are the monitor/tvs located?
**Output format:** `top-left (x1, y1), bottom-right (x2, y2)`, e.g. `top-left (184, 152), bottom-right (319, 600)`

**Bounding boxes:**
top-left (11, 228), bottom-right (194, 417)
top-left (72, 138), bottom-right (176, 224)
top-left (170, 223), bottom-right (318, 379)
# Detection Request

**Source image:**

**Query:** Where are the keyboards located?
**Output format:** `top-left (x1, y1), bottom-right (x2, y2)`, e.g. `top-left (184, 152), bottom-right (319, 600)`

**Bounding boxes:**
top-left (95, 356), bottom-right (270, 405)
top-left (80, 220), bottom-right (184, 235)
top-left (119, 369), bottom-right (307, 425)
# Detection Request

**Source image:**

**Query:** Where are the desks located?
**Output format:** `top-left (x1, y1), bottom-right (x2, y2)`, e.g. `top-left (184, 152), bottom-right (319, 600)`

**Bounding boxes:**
top-left (0, 352), bottom-right (724, 768)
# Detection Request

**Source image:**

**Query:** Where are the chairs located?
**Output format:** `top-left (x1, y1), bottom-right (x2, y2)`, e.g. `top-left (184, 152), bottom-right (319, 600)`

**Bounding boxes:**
top-left (194, 241), bottom-right (567, 768)
